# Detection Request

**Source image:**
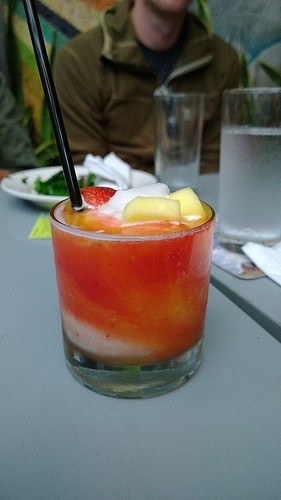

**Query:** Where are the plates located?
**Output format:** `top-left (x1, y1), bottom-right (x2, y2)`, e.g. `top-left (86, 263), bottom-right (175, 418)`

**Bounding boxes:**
top-left (0, 164), bottom-right (158, 209)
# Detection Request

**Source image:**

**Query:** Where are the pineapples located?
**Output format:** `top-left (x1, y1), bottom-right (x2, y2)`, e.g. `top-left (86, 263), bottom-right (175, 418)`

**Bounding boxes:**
top-left (120, 197), bottom-right (181, 224)
top-left (169, 187), bottom-right (205, 214)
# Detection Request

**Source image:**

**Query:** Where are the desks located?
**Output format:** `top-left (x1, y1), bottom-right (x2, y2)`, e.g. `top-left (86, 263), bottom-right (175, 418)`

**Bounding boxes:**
top-left (1, 171), bottom-right (281, 499)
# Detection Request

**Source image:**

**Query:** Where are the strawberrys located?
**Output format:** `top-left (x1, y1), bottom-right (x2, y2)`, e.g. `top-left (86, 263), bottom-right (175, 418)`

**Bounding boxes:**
top-left (78, 186), bottom-right (117, 206)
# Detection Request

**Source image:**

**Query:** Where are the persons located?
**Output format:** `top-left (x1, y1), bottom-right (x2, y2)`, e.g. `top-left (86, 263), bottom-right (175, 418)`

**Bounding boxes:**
top-left (0, 25), bottom-right (37, 180)
top-left (52, 0), bottom-right (244, 176)
top-left (247, 41), bottom-right (281, 121)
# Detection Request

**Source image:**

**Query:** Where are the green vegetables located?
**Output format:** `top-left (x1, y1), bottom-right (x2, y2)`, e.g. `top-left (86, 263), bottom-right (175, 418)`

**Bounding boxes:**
top-left (34, 171), bottom-right (96, 195)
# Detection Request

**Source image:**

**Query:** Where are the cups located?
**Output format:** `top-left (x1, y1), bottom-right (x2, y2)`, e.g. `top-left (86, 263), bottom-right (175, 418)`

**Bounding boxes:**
top-left (51, 193), bottom-right (215, 399)
top-left (153, 92), bottom-right (204, 194)
top-left (217, 87), bottom-right (280, 247)
